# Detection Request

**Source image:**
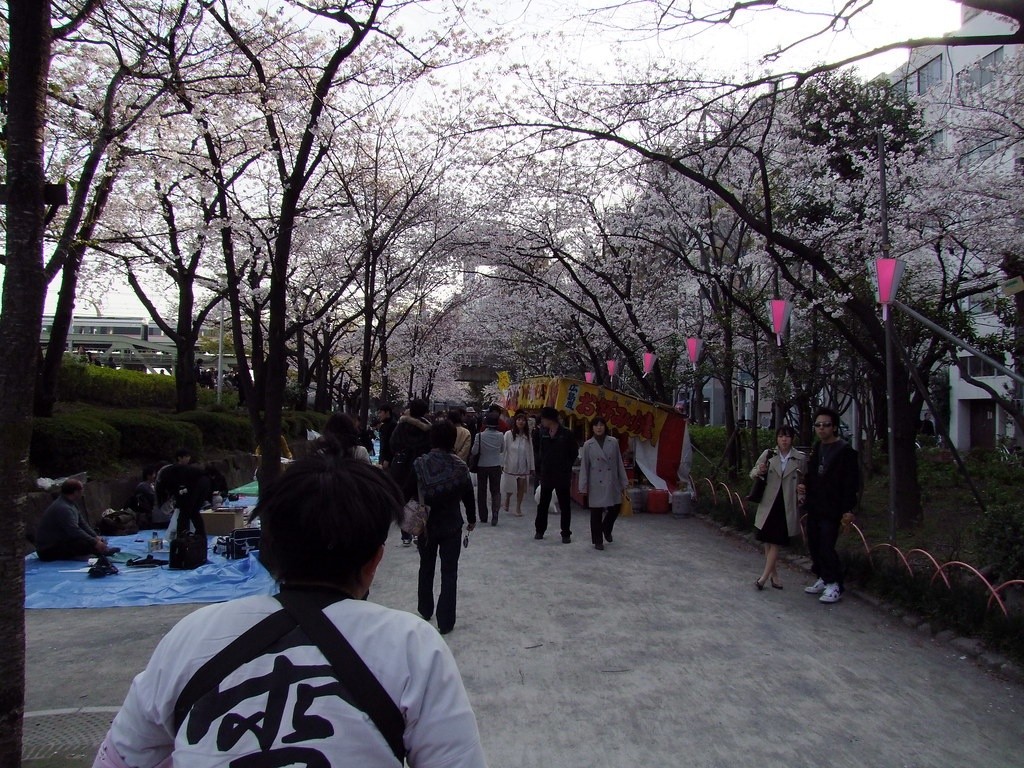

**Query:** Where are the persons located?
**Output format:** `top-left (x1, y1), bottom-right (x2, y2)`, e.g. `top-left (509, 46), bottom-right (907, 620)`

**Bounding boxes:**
top-left (920, 412), bottom-right (934, 448)
top-left (315, 413), bottom-right (372, 465)
top-left (579, 418), bottom-right (629, 549)
top-left (137, 449), bottom-right (228, 527)
top-left (354, 399), bottom-right (541, 546)
top-left (36, 480), bottom-right (121, 562)
top-left (256, 436), bottom-right (292, 470)
top-left (802, 408), bottom-right (859, 602)
top-left (410, 421), bottom-right (476, 634)
top-left (92, 453), bottom-right (487, 768)
top-left (749, 425), bottom-right (807, 590)
top-left (535, 407), bottom-right (578, 544)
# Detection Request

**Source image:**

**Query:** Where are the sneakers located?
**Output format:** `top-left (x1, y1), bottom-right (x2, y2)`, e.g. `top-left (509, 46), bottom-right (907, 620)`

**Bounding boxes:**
top-left (804, 577), bottom-right (827, 593)
top-left (819, 583), bottom-right (844, 603)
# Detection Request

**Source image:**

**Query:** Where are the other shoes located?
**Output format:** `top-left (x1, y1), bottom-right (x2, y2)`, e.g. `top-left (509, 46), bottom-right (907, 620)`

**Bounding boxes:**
top-left (479, 500), bottom-right (571, 545)
top-left (419, 611), bottom-right (430, 620)
top-left (595, 542), bottom-right (604, 550)
top-left (754, 577), bottom-right (784, 590)
top-left (602, 529), bottom-right (613, 542)
top-left (403, 539), bottom-right (411, 546)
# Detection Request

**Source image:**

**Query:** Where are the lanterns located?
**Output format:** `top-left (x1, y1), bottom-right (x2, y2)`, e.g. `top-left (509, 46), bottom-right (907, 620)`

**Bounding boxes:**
top-left (868, 258), bottom-right (906, 321)
top-left (642, 353), bottom-right (656, 378)
top-left (607, 360), bottom-right (617, 382)
top-left (686, 338), bottom-right (703, 371)
top-left (585, 372), bottom-right (595, 383)
top-left (766, 300), bottom-right (793, 346)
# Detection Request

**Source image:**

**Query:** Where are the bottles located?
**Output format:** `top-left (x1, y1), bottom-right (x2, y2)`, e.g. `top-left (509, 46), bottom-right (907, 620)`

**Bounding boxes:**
top-left (153, 532), bottom-right (158, 540)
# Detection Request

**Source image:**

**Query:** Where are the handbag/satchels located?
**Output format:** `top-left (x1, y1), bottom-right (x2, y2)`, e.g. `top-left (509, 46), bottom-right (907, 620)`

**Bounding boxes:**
top-left (88, 555), bottom-right (119, 576)
top-left (745, 449), bottom-right (774, 503)
top-left (534, 483), bottom-right (559, 513)
top-left (619, 489), bottom-right (634, 517)
top-left (99, 507), bottom-right (138, 534)
top-left (468, 453), bottom-right (480, 472)
top-left (391, 450), bottom-right (408, 476)
top-left (399, 500), bottom-right (432, 536)
top-left (214, 528), bottom-right (262, 559)
top-left (169, 530), bottom-right (203, 570)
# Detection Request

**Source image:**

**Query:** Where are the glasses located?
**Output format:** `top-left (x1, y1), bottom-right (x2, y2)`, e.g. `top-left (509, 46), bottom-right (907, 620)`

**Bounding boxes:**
top-left (814, 421), bottom-right (833, 427)
top-left (463, 528), bottom-right (471, 548)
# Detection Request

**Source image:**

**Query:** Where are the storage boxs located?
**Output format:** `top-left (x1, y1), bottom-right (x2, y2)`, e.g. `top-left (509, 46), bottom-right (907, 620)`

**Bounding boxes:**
top-left (201, 508), bottom-right (245, 536)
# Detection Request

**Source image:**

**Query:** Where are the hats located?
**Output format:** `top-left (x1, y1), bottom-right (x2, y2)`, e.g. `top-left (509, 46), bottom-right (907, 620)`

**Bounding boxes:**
top-left (466, 407), bottom-right (476, 412)
top-left (483, 413), bottom-right (499, 427)
top-left (540, 407), bottom-right (559, 421)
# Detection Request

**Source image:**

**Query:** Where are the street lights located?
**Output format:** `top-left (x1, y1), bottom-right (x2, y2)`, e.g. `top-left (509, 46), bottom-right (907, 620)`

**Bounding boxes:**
top-left (215, 272), bottom-right (227, 404)
